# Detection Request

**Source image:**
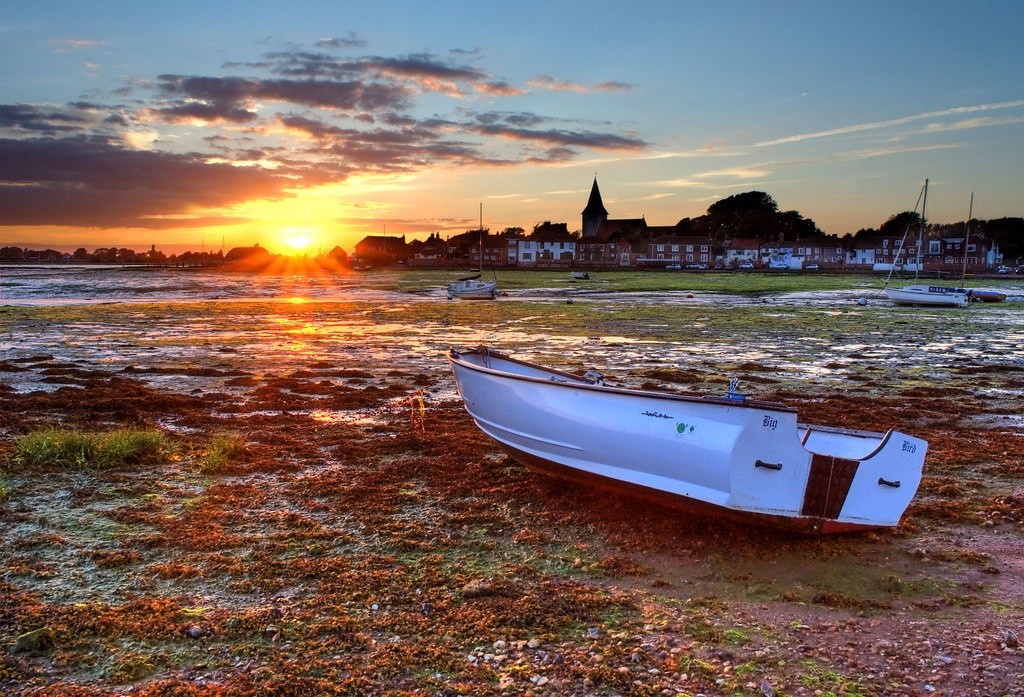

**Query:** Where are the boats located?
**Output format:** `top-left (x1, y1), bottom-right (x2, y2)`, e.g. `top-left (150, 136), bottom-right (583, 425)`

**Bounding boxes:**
top-left (568, 272), bottom-right (590, 279)
top-left (447, 341), bottom-right (932, 540)
top-left (443, 202), bottom-right (500, 302)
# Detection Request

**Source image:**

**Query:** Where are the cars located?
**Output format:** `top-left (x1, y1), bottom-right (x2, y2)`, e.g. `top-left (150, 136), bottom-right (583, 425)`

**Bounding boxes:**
top-left (772, 264), bottom-right (790, 270)
top-left (998, 267), bottom-right (1012, 274)
top-left (665, 264), bottom-right (682, 270)
top-left (739, 263), bottom-right (754, 269)
top-left (698, 263), bottom-right (710, 270)
top-left (725, 263), bottom-right (734, 270)
top-left (1015, 265), bottom-right (1024, 275)
top-left (686, 263), bottom-right (700, 269)
top-left (805, 263), bottom-right (819, 270)
top-left (352, 264), bottom-right (372, 270)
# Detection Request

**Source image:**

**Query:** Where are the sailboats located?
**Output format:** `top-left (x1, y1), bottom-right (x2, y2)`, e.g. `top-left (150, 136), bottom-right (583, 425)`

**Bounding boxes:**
top-left (885, 175), bottom-right (973, 309)
top-left (956, 191), bottom-right (1008, 304)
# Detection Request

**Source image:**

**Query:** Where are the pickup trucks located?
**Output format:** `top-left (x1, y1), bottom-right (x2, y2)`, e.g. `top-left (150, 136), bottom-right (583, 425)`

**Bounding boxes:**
top-left (397, 259), bottom-right (404, 264)
